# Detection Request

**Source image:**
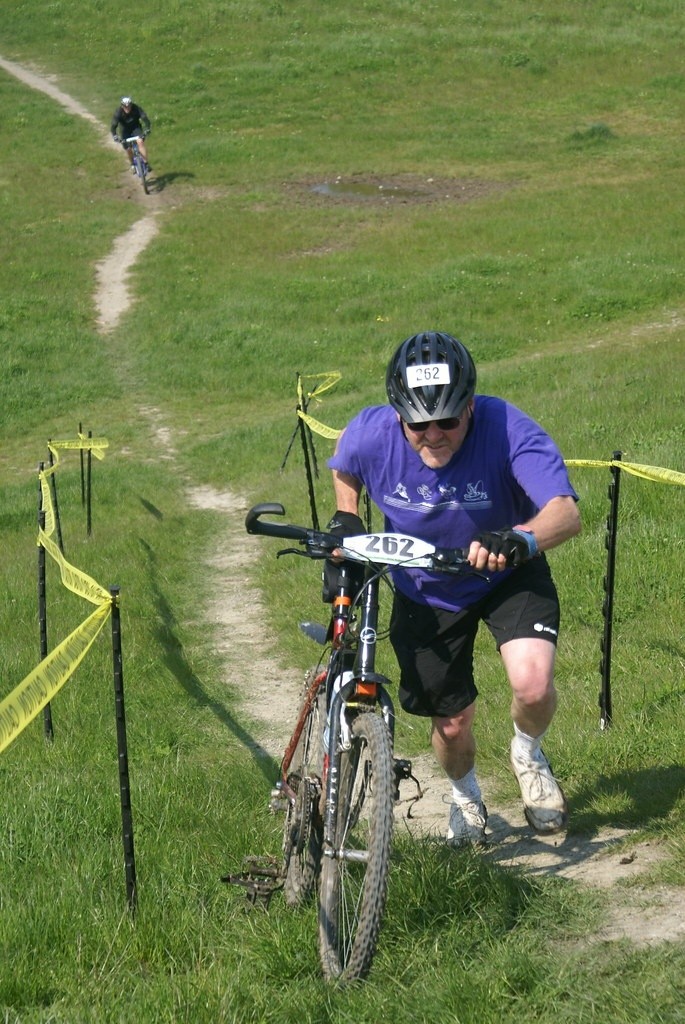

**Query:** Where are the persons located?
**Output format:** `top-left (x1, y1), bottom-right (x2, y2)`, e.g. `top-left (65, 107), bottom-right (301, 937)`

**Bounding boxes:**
top-left (111, 98), bottom-right (152, 174)
top-left (323, 333), bottom-right (582, 845)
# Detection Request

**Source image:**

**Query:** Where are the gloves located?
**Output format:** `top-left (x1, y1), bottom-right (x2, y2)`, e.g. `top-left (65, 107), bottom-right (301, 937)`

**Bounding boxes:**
top-left (144, 128), bottom-right (150, 136)
top-left (113, 136), bottom-right (119, 143)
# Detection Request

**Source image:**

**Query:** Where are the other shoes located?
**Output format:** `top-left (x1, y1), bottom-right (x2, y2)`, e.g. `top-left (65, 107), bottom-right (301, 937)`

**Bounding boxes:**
top-left (130, 165), bottom-right (135, 175)
top-left (143, 161), bottom-right (151, 172)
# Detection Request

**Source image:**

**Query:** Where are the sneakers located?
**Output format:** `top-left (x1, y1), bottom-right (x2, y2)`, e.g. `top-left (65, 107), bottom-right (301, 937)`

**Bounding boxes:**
top-left (442, 794), bottom-right (487, 851)
top-left (509, 746), bottom-right (568, 831)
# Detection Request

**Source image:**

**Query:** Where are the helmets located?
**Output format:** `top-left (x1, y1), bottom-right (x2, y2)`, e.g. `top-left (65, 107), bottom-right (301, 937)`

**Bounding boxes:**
top-left (386, 330), bottom-right (476, 424)
top-left (121, 98), bottom-right (132, 107)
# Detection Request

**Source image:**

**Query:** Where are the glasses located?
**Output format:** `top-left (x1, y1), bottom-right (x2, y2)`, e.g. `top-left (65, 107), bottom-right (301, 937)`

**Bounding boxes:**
top-left (407, 414), bottom-right (464, 432)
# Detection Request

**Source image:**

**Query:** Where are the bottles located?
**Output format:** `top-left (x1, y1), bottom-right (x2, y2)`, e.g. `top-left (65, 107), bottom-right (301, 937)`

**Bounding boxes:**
top-left (323, 670), bottom-right (355, 753)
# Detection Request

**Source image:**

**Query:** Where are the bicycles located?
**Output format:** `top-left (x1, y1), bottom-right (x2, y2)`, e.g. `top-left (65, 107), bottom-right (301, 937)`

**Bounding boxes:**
top-left (219, 500), bottom-right (522, 991)
top-left (112, 132), bottom-right (151, 196)
